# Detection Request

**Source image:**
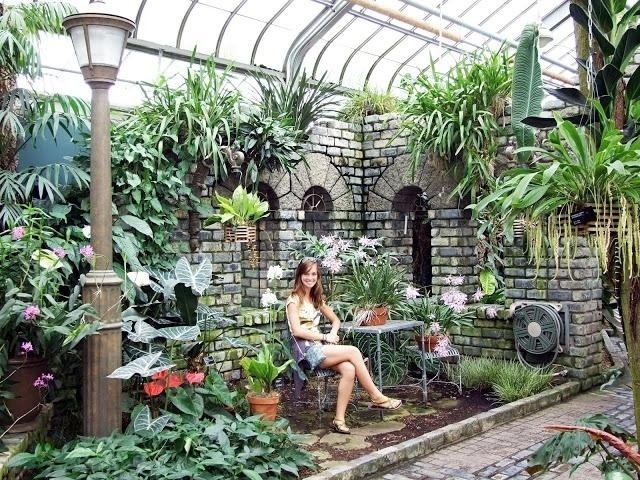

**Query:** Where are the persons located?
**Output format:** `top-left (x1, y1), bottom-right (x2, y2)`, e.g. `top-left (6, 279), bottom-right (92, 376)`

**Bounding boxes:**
top-left (284, 256), bottom-right (404, 434)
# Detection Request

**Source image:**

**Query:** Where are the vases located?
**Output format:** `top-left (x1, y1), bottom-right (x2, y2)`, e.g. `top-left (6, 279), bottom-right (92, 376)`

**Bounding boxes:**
top-left (5, 357), bottom-right (46, 424)
top-left (416, 333), bottom-right (439, 353)
top-left (352, 302), bottom-right (388, 326)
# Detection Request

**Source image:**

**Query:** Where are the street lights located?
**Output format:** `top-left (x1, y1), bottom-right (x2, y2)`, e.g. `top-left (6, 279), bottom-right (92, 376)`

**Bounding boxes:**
top-left (61, 0), bottom-right (138, 438)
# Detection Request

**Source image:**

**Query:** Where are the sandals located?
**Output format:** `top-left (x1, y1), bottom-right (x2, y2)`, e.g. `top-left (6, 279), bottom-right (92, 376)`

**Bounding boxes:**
top-left (332, 417), bottom-right (350, 433)
top-left (368, 397), bottom-right (402, 410)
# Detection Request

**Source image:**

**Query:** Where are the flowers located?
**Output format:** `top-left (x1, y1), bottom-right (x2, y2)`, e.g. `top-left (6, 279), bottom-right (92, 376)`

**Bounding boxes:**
top-left (405, 274), bottom-right (487, 336)
top-left (318, 233), bottom-right (406, 308)
top-left (0, 201), bottom-right (103, 357)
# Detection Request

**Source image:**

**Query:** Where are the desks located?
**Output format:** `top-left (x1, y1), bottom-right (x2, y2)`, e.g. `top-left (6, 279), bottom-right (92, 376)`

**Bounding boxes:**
top-left (319, 320), bottom-right (425, 421)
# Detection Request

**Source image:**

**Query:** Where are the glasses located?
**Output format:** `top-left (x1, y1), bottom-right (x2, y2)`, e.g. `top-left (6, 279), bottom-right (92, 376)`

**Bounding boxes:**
top-left (299, 257), bottom-right (317, 265)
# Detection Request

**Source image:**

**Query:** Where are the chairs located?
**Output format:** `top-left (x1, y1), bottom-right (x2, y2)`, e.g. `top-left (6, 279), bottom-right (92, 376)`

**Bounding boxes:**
top-left (285, 310), bottom-right (337, 421)
top-left (403, 325), bottom-right (463, 401)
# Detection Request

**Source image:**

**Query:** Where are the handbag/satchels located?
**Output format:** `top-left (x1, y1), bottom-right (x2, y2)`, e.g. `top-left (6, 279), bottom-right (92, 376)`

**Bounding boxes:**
top-left (305, 345), bottom-right (326, 370)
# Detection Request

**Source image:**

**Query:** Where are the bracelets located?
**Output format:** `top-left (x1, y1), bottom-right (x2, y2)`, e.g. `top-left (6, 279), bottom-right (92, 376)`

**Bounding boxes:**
top-left (322, 333), bottom-right (327, 342)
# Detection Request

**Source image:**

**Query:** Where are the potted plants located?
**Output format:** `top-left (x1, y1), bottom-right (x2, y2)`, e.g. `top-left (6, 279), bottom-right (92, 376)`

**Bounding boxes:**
top-left (463, 97), bottom-right (640, 282)
top-left (238, 338), bottom-right (295, 422)
top-left (202, 185), bottom-right (272, 242)
